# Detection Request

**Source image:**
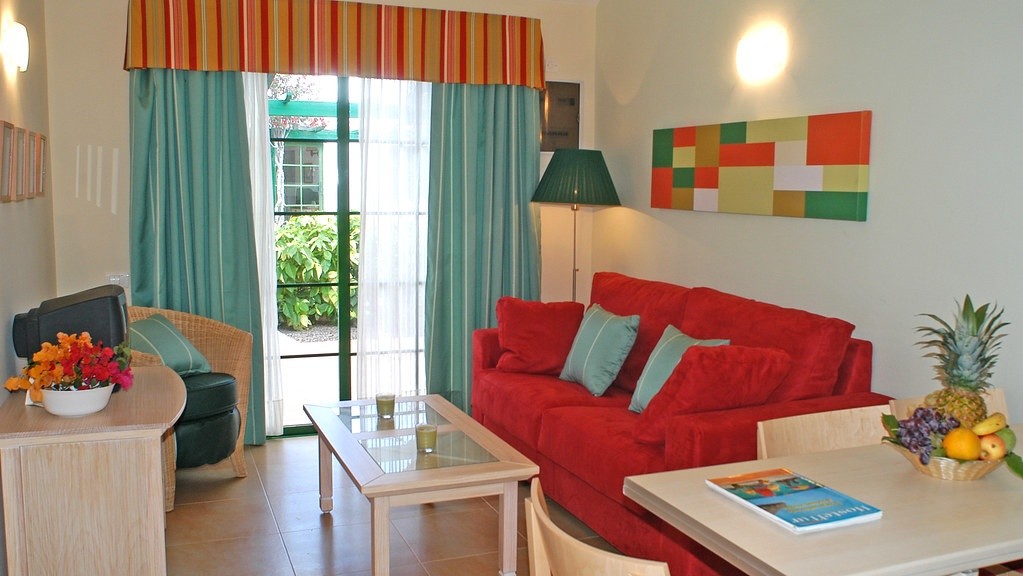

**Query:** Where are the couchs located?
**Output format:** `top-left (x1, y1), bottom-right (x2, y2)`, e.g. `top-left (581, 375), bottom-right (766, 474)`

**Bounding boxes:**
top-left (470, 271), bottom-right (898, 576)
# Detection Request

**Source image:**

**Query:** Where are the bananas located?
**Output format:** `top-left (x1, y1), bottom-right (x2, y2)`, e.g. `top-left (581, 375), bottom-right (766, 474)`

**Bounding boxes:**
top-left (972, 413), bottom-right (1006, 436)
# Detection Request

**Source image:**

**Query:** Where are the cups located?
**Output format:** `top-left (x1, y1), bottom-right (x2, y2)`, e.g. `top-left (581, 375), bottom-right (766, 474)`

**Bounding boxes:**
top-left (376, 393), bottom-right (395, 417)
top-left (416, 424), bottom-right (437, 454)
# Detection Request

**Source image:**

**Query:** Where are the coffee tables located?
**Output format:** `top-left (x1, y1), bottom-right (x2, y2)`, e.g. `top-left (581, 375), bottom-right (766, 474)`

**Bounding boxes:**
top-left (305, 391), bottom-right (541, 576)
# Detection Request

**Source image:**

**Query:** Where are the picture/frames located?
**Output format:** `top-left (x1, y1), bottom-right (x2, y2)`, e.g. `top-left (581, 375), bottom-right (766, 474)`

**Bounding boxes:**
top-left (26, 129), bottom-right (37, 200)
top-left (12, 127), bottom-right (27, 202)
top-left (0, 120), bottom-right (14, 204)
top-left (37, 133), bottom-right (48, 198)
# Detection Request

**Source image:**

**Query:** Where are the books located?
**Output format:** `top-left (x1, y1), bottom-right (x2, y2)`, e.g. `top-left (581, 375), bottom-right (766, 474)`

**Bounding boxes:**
top-left (705, 468), bottom-right (883, 534)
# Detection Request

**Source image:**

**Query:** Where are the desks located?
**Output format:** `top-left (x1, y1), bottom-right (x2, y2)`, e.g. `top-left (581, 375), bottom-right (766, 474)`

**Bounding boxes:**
top-left (622, 421), bottom-right (1023, 576)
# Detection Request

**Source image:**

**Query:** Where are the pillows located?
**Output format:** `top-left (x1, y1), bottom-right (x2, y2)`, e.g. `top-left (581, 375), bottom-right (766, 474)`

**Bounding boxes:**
top-left (129, 312), bottom-right (214, 377)
top-left (558, 303), bottom-right (641, 397)
top-left (626, 324), bottom-right (731, 414)
top-left (631, 344), bottom-right (791, 449)
top-left (495, 297), bottom-right (584, 376)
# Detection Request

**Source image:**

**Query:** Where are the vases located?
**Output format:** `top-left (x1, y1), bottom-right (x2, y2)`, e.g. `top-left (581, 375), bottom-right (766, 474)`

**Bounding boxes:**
top-left (39, 383), bottom-right (115, 417)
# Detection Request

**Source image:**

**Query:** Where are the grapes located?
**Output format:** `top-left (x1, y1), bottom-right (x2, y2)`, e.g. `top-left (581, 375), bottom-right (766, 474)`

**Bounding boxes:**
top-left (913, 293), bottom-right (1010, 425)
top-left (897, 407), bottom-right (961, 464)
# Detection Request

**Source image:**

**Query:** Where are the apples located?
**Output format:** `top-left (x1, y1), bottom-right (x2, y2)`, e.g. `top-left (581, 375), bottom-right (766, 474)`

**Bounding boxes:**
top-left (979, 434), bottom-right (1006, 461)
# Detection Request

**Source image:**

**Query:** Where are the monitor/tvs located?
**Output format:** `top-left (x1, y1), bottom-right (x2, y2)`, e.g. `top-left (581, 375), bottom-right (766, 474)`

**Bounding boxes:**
top-left (13, 284), bottom-right (127, 392)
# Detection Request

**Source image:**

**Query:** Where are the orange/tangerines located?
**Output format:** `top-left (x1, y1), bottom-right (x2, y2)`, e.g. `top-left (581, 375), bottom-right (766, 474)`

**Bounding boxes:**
top-left (943, 427), bottom-right (981, 461)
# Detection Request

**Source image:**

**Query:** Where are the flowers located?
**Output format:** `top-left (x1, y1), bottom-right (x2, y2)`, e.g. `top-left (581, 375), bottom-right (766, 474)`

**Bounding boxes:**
top-left (4, 329), bottom-right (133, 403)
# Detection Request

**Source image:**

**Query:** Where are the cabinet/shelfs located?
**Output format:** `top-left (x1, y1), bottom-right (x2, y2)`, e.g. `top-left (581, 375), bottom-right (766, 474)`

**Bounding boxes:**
top-left (0, 363), bottom-right (188, 576)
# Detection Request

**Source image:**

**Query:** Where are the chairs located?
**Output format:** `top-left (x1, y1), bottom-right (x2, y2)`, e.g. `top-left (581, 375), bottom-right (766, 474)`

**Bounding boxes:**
top-left (756, 404), bottom-right (893, 461)
top-left (890, 387), bottom-right (1010, 430)
top-left (126, 304), bottom-right (254, 514)
top-left (522, 477), bottom-right (673, 576)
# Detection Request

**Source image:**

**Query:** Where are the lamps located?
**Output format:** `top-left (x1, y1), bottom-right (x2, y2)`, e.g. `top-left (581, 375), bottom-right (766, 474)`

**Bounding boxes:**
top-left (9, 20), bottom-right (31, 74)
top-left (529, 148), bottom-right (623, 302)
